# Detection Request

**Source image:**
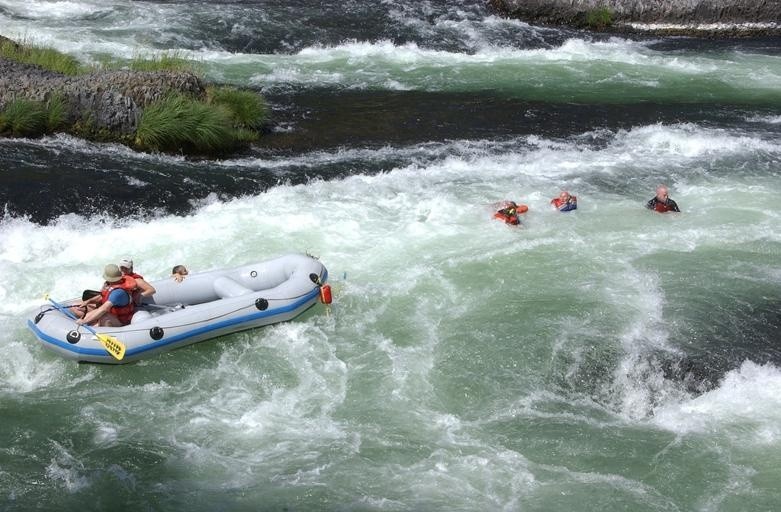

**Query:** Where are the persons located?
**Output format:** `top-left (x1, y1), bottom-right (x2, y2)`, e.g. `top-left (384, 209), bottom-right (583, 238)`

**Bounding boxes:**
top-left (645, 187), bottom-right (680, 213)
top-left (76, 263), bottom-right (135, 328)
top-left (71, 256), bottom-right (157, 317)
top-left (170, 265), bottom-right (188, 283)
top-left (551, 192), bottom-right (580, 211)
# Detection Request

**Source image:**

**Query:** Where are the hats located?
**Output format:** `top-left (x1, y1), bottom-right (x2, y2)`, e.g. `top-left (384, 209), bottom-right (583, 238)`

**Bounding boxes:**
top-left (102, 264), bottom-right (122, 282)
top-left (118, 257), bottom-right (132, 269)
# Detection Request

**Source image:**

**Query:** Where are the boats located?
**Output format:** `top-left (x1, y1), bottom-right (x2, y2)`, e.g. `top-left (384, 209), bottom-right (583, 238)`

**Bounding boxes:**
top-left (27, 253), bottom-right (327, 364)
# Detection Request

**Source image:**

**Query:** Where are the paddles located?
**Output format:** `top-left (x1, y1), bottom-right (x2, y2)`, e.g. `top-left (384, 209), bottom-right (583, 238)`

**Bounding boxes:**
top-left (43, 293), bottom-right (126, 361)
top-left (82, 289), bottom-right (181, 308)
